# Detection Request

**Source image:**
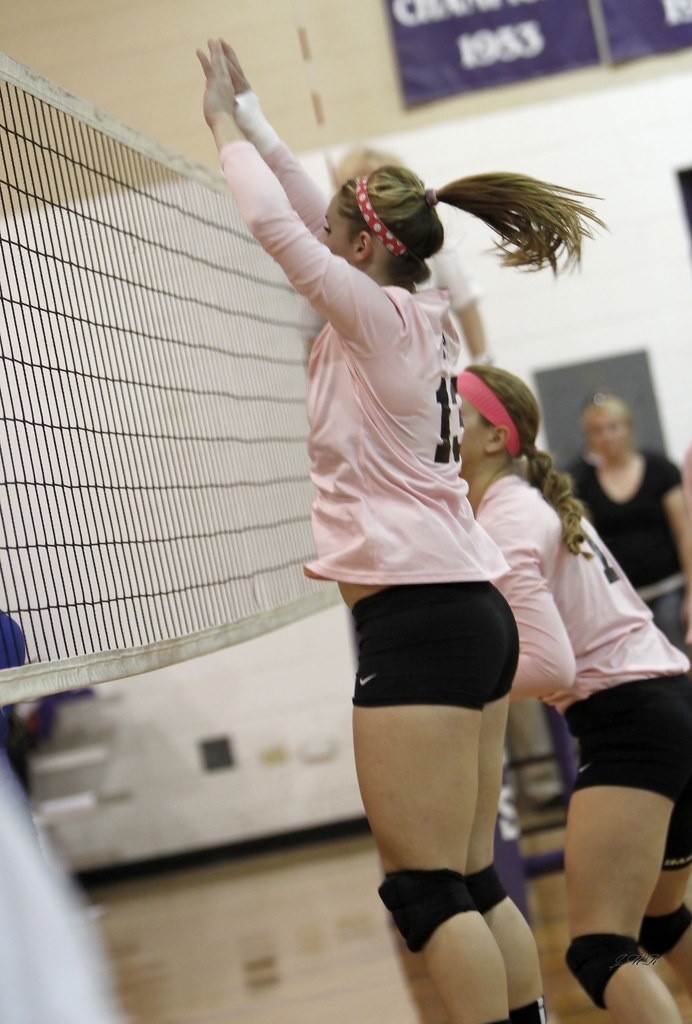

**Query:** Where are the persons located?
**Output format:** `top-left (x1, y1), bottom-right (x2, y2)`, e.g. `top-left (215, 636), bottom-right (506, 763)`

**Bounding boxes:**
top-left (195, 37), bottom-right (599, 1023)
top-left (457, 365), bottom-right (692, 1024)
top-left (0, 610), bottom-right (127, 1024)
top-left (571, 394), bottom-right (692, 650)
